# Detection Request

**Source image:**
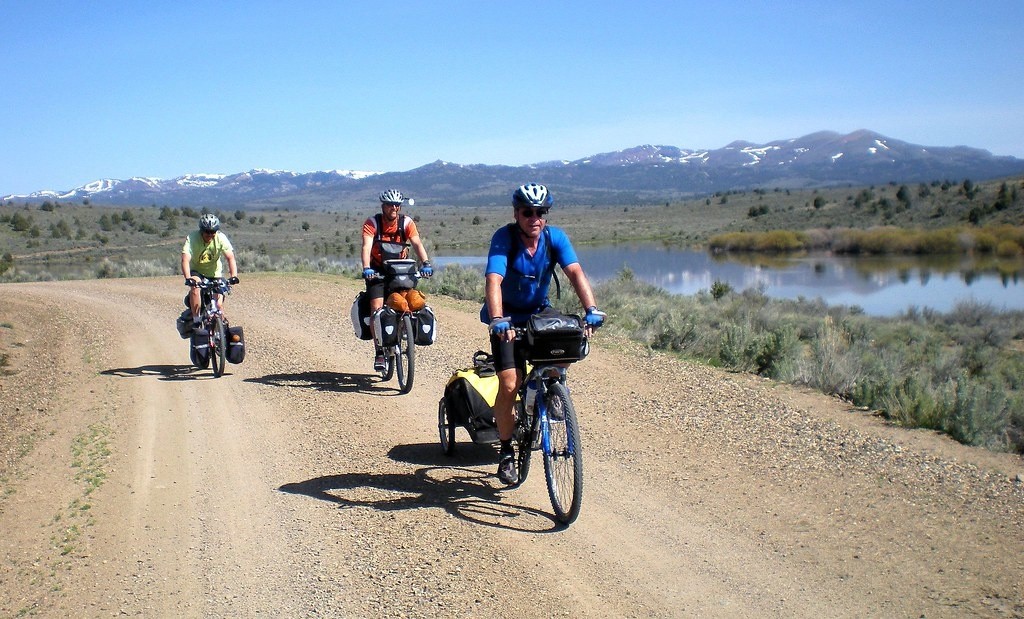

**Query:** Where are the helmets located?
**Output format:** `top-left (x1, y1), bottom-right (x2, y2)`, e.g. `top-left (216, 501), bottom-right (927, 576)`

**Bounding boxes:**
top-left (379, 189), bottom-right (404, 203)
top-left (199, 213), bottom-right (220, 232)
top-left (512, 182), bottom-right (553, 209)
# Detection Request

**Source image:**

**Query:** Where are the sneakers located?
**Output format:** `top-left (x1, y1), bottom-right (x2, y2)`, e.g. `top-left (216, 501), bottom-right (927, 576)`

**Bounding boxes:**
top-left (546, 389), bottom-right (564, 421)
top-left (374, 357), bottom-right (386, 370)
top-left (497, 445), bottom-right (518, 484)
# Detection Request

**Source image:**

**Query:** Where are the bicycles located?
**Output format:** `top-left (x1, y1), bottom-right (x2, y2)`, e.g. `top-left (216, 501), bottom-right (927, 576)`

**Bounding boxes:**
top-left (176, 276), bottom-right (246, 378)
top-left (437, 315), bottom-right (605, 525)
top-left (350, 269), bottom-right (438, 394)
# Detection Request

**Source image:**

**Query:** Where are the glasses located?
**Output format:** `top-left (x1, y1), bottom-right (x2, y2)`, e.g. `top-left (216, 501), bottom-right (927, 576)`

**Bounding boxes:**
top-left (201, 226), bottom-right (216, 234)
top-left (521, 209), bottom-right (547, 218)
top-left (383, 202), bottom-right (401, 206)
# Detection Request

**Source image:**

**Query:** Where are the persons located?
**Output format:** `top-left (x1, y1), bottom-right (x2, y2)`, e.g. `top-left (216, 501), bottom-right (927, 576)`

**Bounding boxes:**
top-left (484, 183), bottom-right (604, 484)
top-left (361, 188), bottom-right (433, 370)
top-left (181, 214), bottom-right (239, 331)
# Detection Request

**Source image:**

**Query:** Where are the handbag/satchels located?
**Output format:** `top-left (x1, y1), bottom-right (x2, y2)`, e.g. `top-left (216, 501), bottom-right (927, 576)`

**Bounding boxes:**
top-left (524, 313), bottom-right (590, 365)
top-left (411, 307), bottom-right (437, 346)
top-left (190, 327), bottom-right (209, 369)
top-left (177, 309), bottom-right (194, 339)
top-left (444, 350), bottom-right (535, 430)
top-left (351, 291), bottom-right (373, 340)
top-left (383, 258), bottom-right (417, 290)
top-left (224, 323), bottom-right (245, 364)
top-left (370, 303), bottom-right (400, 347)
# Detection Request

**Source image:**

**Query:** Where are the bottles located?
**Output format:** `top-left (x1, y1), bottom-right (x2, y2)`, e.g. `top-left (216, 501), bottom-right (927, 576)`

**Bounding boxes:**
top-left (525, 380), bottom-right (538, 415)
